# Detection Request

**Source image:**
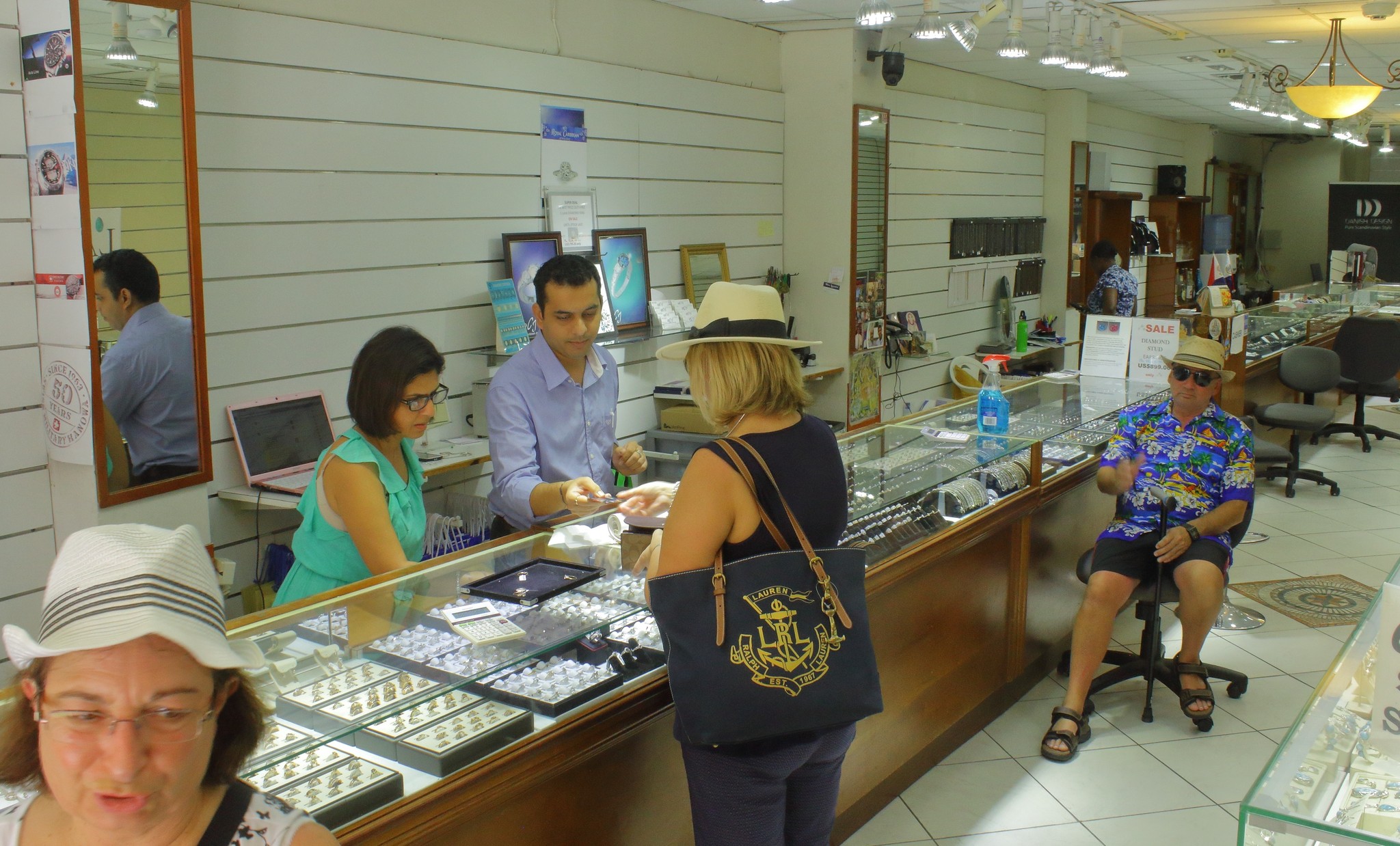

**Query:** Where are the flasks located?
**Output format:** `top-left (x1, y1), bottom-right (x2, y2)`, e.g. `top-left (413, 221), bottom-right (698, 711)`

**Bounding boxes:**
top-left (1016, 310), bottom-right (1028, 352)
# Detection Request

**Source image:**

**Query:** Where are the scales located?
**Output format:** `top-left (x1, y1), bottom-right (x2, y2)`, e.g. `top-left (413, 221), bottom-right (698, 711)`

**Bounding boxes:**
top-left (979, 341), bottom-right (1012, 354)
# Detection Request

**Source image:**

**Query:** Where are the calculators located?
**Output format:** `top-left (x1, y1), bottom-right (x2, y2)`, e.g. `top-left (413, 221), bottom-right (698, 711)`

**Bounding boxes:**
top-left (441, 601), bottom-right (527, 644)
top-left (1043, 368), bottom-right (1080, 380)
top-left (1274, 297), bottom-right (1303, 303)
top-left (920, 426), bottom-right (971, 443)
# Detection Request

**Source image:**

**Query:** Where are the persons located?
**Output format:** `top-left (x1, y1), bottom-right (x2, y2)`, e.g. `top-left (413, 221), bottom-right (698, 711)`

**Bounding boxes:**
top-left (633, 280), bottom-right (883, 846)
top-left (484, 255), bottom-right (648, 573)
top-left (99, 403), bottom-right (130, 492)
top-left (1075, 239), bottom-right (1138, 317)
top-left (0, 522), bottom-right (337, 846)
top-left (270, 325), bottom-right (462, 608)
top-left (92, 248), bottom-right (201, 487)
top-left (1040, 335), bottom-right (1255, 762)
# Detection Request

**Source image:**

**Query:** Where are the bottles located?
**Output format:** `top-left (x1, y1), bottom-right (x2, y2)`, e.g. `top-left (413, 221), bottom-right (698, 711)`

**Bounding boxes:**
top-left (1175, 266), bottom-right (1203, 305)
top-left (1202, 214), bottom-right (1233, 253)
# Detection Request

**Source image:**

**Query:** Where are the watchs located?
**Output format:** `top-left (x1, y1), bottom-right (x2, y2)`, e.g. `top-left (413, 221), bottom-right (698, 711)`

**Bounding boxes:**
top-left (1181, 523), bottom-right (1200, 541)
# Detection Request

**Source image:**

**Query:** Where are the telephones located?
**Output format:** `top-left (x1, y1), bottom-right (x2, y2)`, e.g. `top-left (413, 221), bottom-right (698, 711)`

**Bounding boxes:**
top-left (886, 319), bottom-right (907, 336)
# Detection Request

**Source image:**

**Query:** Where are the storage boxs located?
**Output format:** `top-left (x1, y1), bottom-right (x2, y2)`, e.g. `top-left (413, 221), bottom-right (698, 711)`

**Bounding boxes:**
top-left (646, 403), bottom-right (728, 482)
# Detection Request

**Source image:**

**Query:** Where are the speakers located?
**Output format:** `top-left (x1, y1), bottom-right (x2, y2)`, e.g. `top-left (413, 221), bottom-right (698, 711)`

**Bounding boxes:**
top-left (1157, 165), bottom-right (1187, 195)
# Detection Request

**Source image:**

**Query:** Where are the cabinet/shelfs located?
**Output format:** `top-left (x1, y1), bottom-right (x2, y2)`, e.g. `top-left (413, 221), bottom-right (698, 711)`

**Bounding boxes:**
top-left (1146, 195), bottom-right (1211, 319)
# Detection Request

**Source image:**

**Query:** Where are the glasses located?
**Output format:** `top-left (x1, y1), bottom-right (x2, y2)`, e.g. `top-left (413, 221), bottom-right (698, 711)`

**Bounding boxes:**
top-left (35, 685), bottom-right (219, 744)
top-left (1170, 366), bottom-right (1222, 387)
top-left (398, 382), bottom-right (449, 412)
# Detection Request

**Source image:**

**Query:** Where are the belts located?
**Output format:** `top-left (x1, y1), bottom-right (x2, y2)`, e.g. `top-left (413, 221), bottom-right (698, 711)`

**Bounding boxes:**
top-left (495, 514), bottom-right (518, 535)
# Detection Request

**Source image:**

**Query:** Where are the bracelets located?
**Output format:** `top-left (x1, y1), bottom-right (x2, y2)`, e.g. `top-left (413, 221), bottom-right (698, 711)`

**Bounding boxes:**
top-left (559, 481), bottom-right (567, 505)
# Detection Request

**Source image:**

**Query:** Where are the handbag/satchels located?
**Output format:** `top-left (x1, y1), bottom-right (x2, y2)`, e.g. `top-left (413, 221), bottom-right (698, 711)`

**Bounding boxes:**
top-left (647, 436), bottom-right (884, 750)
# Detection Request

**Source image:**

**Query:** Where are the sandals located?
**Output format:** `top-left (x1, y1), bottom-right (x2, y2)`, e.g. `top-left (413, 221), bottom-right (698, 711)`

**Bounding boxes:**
top-left (1174, 651), bottom-right (1215, 719)
top-left (1041, 705), bottom-right (1091, 761)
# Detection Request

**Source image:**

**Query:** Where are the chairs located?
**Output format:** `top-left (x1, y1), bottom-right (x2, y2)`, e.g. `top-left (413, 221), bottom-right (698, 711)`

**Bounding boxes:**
top-left (1309, 315), bottom-right (1400, 452)
top-left (950, 356), bottom-right (1021, 397)
top-left (1236, 416), bottom-right (1293, 543)
top-left (1057, 488), bottom-right (1253, 721)
top-left (1255, 345), bottom-right (1341, 496)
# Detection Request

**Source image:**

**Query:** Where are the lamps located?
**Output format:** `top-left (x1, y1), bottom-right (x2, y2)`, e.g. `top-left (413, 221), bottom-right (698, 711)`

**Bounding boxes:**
top-left (104, 1), bottom-right (178, 108)
top-left (1228, 17), bottom-right (1400, 152)
top-left (855, 0), bottom-right (1129, 78)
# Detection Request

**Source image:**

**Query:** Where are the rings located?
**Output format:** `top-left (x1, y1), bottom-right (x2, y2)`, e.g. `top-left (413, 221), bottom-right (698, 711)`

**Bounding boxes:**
top-left (634, 449), bottom-right (642, 458)
top-left (575, 495), bottom-right (579, 505)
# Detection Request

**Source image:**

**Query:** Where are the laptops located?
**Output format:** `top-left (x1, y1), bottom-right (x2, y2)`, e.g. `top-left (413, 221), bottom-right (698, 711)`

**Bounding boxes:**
top-left (222, 391), bottom-right (335, 499)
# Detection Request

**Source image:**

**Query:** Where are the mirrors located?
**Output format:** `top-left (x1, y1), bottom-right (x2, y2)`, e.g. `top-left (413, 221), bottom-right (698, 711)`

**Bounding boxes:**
top-left (1067, 141), bottom-right (1089, 309)
top-left (849, 104), bottom-right (890, 357)
top-left (680, 242), bottom-right (730, 311)
top-left (71, 0), bottom-right (213, 507)
top-left (1202, 162), bottom-right (1214, 255)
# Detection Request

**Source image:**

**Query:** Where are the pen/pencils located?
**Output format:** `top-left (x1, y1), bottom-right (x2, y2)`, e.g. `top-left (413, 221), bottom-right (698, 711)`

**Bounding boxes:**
top-left (1040, 314), bottom-right (1058, 328)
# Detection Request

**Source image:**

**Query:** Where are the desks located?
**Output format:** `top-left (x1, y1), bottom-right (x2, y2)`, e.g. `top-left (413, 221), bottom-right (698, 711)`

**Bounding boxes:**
top-left (977, 340), bottom-right (1084, 360)
top-left (653, 364), bottom-right (844, 400)
top-left (217, 439), bottom-right (492, 508)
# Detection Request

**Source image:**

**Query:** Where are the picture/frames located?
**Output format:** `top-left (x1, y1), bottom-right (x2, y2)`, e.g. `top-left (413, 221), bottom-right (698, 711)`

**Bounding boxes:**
top-left (592, 227), bottom-right (652, 329)
top-left (590, 260), bottom-right (619, 338)
top-left (502, 231), bottom-right (564, 341)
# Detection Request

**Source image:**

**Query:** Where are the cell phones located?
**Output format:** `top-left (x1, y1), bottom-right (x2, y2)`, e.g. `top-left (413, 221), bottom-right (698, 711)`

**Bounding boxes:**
top-left (1070, 301), bottom-right (1087, 313)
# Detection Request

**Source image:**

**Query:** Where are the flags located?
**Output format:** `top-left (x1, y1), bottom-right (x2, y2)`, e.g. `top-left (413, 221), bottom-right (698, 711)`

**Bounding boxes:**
top-left (1208, 253), bottom-right (1234, 291)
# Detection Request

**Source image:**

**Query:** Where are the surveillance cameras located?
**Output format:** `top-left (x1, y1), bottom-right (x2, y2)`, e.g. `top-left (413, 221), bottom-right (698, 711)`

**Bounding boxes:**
top-left (1212, 128), bottom-right (1220, 135)
top-left (882, 51), bottom-right (904, 86)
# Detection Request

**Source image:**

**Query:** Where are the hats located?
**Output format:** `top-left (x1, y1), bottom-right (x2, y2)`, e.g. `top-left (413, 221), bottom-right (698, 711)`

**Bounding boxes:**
top-left (3, 523), bottom-right (265, 669)
top-left (655, 282), bottom-right (823, 361)
top-left (1161, 336), bottom-right (1235, 384)
